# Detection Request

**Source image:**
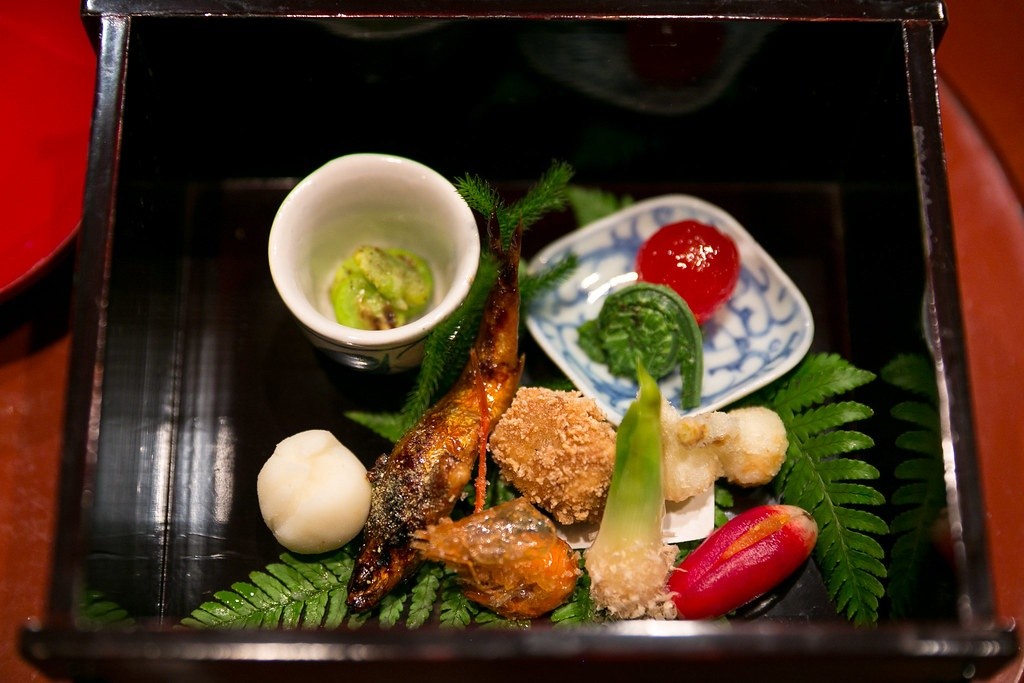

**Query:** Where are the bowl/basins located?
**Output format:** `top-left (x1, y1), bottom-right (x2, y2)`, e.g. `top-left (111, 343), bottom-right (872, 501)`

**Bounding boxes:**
top-left (271, 153), bottom-right (481, 377)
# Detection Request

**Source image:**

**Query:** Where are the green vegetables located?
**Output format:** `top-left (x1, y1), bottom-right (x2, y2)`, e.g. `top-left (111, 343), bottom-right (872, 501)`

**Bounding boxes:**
top-left (176, 163), bottom-right (888, 641)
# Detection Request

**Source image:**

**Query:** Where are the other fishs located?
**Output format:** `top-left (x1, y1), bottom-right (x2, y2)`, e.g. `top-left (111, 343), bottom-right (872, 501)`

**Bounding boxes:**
top-left (344, 208), bottom-right (523, 615)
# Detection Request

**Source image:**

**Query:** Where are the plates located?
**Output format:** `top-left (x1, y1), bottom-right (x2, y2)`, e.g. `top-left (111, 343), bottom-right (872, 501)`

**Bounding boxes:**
top-left (0, 0), bottom-right (100, 295)
top-left (525, 196), bottom-right (815, 430)
